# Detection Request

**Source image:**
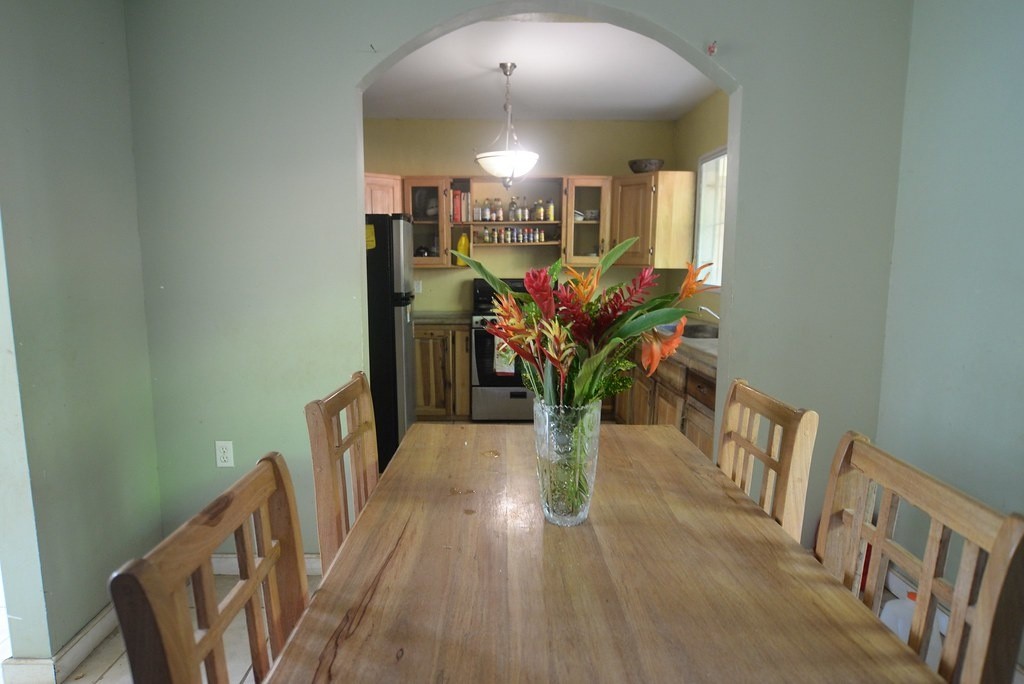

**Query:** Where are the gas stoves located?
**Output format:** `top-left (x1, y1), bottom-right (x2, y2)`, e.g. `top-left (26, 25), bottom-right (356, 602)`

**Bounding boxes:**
top-left (472, 279), bottom-right (562, 328)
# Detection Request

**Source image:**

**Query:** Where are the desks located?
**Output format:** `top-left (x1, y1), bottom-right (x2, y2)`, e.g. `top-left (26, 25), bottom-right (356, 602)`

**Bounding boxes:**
top-left (260, 422), bottom-right (947, 684)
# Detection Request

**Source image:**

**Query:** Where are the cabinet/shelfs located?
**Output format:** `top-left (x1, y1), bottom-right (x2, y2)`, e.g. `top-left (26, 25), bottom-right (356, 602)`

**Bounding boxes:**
top-left (413, 323), bottom-right (717, 462)
top-left (364, 171), bottom-right (696, 270)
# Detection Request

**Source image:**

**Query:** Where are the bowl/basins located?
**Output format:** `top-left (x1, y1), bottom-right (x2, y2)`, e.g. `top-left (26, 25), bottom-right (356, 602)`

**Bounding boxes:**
top-left (627, 158), bottom-right (665, 174)
top-left (588, 253), bottom-right (597, 256)
top-left (575, 209), bottom-right (599, 221)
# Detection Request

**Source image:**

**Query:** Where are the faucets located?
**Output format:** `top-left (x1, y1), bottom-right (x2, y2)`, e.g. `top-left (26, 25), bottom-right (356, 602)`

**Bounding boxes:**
top-left (697, 305), bottom-right (720, 320)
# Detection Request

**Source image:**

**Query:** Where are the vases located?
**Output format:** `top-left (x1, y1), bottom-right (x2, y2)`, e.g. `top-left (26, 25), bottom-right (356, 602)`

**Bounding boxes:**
top-left (532, 396), bottom-right (602, 530)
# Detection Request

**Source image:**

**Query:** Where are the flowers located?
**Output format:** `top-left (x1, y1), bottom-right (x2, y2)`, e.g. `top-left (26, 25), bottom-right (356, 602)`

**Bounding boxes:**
top-left (446, 237), bottom-right (720, 516)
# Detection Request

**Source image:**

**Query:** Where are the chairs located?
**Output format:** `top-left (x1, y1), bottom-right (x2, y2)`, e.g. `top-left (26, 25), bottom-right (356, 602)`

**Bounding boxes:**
top-left (813, 430), bottom-right (1024, 684)
top-left (105, 451), bottom-right (309, 684)
top-left (304, 370), bottom-right (381, 578)
top-left (717, 379), bottom-right (821, 544)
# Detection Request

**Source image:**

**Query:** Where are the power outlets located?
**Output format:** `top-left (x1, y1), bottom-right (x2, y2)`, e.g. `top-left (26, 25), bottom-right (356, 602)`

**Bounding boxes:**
top-left (414, 280), bottom-right (423, 294)
top-left (215, 441), bottom-right (234, 467)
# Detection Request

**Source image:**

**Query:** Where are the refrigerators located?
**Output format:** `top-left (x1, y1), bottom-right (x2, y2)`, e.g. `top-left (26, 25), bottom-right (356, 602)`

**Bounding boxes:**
top-left (365, 213), bottom-right (415, 454)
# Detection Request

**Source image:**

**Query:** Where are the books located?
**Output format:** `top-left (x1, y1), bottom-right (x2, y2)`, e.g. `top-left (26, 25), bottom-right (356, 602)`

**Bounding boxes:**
top-left (450, 189), bottom-right (470, 222)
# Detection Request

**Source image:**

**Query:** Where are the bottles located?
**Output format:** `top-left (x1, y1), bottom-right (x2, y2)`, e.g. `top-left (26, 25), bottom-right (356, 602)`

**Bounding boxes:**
top-left (457, 233), bottom-right (469, 266)
top-left (880, 591), bottom-right (943, 674)
top-left (484, 227), bottom-right (544, 243)
top-left (473, 196), bottom-right (555, 222)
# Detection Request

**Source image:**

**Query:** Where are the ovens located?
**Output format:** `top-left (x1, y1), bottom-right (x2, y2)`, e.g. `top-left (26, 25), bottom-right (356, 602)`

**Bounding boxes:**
top-left (472, 327), bottom-right (535, 422)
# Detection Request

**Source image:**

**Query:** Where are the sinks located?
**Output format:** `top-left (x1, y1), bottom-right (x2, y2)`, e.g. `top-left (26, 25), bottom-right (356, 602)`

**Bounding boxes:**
top-left (656, 322), bottom-right (719, 339)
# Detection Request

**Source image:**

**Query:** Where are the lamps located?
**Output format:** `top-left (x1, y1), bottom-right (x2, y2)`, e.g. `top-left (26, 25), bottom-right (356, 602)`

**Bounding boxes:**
top-left (472, 62), bottom-right (547, 192)
